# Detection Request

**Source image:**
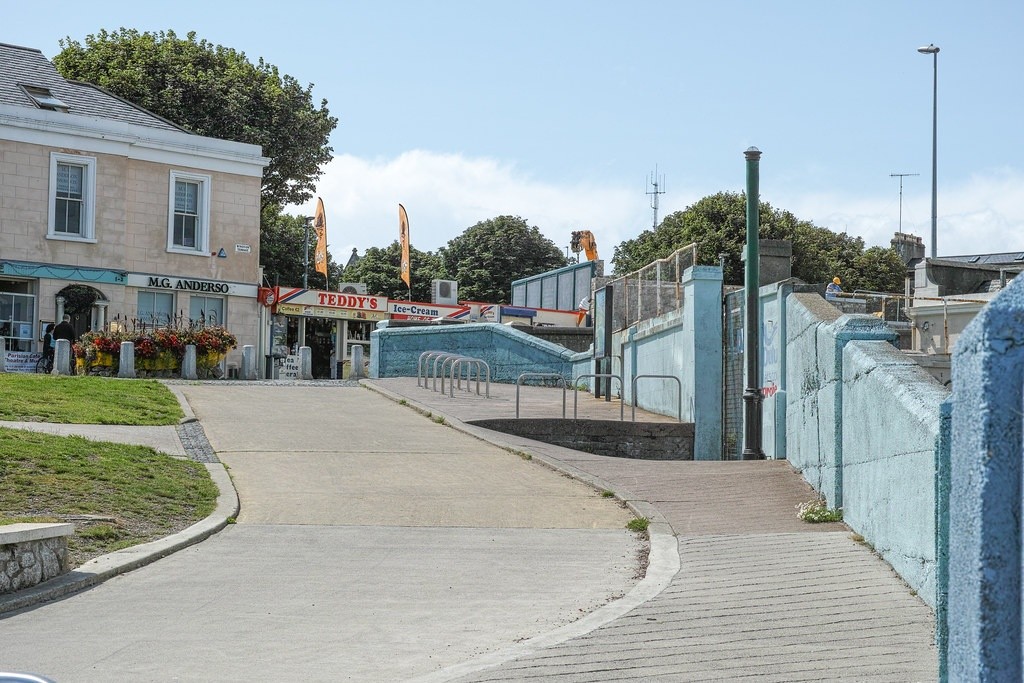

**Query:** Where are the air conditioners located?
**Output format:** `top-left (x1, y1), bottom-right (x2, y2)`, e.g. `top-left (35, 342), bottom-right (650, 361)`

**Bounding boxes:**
top-left (431, 279), bottom-right (459, 306)
top-left (338, 282), bottom-right (369, 296)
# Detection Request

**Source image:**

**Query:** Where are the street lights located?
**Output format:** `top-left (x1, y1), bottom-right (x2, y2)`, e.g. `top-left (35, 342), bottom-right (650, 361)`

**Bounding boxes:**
top-left (916, 42), bottom-right (941, 258)
top-left (741, 146), bottom-right (763, 459)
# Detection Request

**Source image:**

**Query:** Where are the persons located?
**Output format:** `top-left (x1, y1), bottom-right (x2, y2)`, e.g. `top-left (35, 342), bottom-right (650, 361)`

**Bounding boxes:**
top-left (53, 314), bottom-right (75, 345)
top-left (826, 277), bottom-right (843, 298)
top-left (43, 324), bottom-right (55, 374)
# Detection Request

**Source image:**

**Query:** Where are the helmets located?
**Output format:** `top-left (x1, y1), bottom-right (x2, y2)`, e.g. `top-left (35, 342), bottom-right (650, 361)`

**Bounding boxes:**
top-left (833, 277), bottom-right (841, 285)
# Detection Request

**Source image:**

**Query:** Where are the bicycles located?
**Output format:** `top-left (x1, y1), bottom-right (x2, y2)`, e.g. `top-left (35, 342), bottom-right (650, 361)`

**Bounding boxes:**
top-left (36, 349), bottom-right (54, 375)
